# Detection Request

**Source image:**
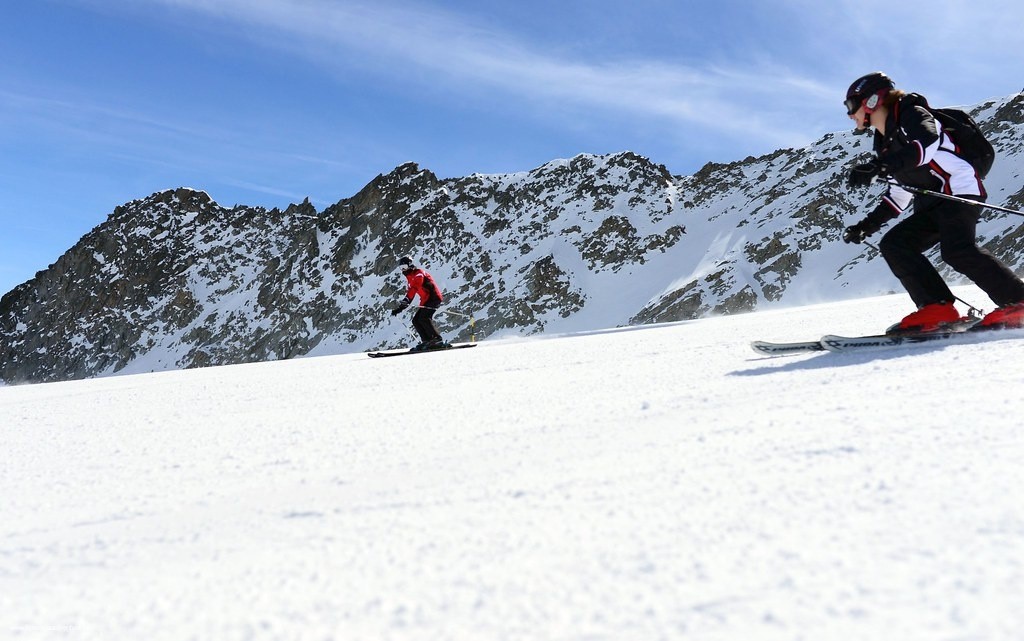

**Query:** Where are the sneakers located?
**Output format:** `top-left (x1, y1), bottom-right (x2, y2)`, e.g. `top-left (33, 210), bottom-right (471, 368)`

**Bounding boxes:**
top-left (901, 303), bottom-right (960, 332)
top-left (982, 301), bottom-right (1024, 326)
top-left (416, 335), bottom-right (443, 349)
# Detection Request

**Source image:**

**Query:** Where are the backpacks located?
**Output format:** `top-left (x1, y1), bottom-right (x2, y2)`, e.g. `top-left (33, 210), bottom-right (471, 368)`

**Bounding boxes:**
top-left (894, 93), bottom-right (995, 181)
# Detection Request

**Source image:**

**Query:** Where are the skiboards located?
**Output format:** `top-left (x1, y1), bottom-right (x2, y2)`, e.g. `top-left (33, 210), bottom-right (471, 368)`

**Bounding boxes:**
top-left (363, 342), bottom-right (480, 360)
top-left (753, 315), bottom-right (1019, 353)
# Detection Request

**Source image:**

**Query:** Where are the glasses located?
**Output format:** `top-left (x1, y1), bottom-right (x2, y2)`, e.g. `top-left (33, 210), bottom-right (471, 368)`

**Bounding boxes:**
top-left (399, 264), bottom-right (408, 269)
top-left (844, 79), bottom-right (891, 115)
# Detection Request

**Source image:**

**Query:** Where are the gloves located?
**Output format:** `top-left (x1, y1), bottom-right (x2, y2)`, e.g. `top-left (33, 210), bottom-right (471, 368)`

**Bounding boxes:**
top-left (848, 144), bottom-right (921, 189)
top-left (843, 202), bottom-right (894, 244)
top-left (399, 298), bottom-right (410, 308)
top-left (392, 308), bottom-right (402, 315)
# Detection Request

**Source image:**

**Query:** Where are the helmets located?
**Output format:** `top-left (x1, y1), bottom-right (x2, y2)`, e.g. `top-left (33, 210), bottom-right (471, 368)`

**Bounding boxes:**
top-left (399, 257), bottom-right (412, 265)
top-left (847, 72), bottom-right (894, 114)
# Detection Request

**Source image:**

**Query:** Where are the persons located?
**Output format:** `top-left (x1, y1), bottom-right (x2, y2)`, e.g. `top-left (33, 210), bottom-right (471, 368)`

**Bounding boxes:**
top-left (842, 71), bottom-right (1024, 336)
top-left (391, 257), bottom-right (443, 350)
